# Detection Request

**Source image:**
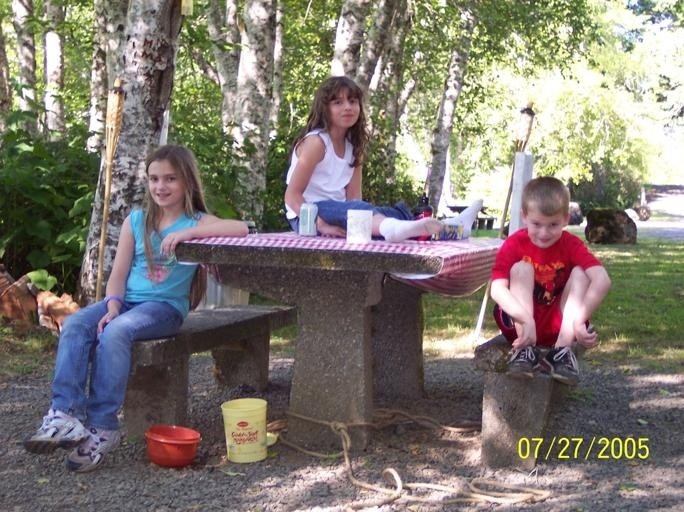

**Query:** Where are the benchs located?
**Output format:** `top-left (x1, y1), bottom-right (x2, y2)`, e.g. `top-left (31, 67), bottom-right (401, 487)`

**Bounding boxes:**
top-left (437, 216), bottom-right (454, 221)
top-left (90, 305), bottom-right (297, 442)
top-left (477, 218), bottom-right (497, 229)
top-left (474, 324), bottom-right (595, 471)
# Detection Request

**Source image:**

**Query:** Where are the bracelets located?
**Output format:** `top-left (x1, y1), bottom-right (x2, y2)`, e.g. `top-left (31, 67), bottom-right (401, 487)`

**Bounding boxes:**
top-left (103, 295), bottom-right (122, 311)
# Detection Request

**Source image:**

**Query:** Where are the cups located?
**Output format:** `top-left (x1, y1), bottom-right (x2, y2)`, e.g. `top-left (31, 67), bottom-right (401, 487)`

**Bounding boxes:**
top-left (346, 209), bottom-right (373, 244)
top-left (413, 192), bottom-right (433, 241)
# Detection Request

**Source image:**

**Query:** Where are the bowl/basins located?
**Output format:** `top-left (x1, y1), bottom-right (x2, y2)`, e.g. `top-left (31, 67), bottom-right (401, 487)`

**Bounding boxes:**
top-left (142, 425), bottom-right (200, 467)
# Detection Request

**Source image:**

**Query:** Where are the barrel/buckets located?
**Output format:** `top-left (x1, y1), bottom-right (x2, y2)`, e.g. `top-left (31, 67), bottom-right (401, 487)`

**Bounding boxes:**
top-left (220, 398), bottom-right (269, 464)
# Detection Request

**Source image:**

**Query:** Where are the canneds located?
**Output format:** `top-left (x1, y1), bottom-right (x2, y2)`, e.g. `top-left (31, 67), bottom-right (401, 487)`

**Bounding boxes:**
top-left (298, 203), bottom-right (318, 237)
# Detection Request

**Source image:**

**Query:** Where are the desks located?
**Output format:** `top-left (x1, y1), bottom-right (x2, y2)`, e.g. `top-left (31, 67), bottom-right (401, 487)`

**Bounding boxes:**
top-left (174, 230), bottom-right (505, 451)
top-left (447, 206), bottom-right (476, 229)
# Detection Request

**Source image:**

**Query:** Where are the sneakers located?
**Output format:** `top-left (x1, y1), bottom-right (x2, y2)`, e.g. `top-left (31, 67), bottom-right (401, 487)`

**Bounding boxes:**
top-left (508, 343), bottom-right (541, 380)
top-left (542, 344), bottom-right (580, 386)
top-left (23, 410), bottom-right (88, 455)
top-left (66, 423), bottom-right (125, 473)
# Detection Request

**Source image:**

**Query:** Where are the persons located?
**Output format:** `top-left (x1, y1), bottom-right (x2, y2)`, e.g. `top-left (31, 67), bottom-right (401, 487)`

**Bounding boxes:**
top-left (22, 142), bottom-right (248, 473)
top-left (486, 175), bottom-right (612, 385)
top-left (281, 76), bottom-right (486, 245)
